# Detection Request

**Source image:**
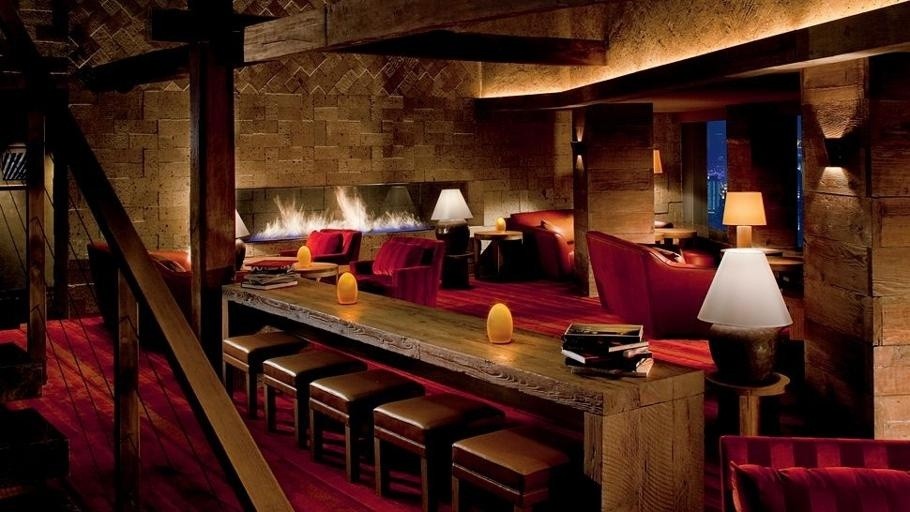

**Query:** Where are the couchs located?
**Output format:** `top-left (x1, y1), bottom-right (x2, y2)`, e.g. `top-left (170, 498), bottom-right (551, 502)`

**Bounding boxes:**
top-left (87, 243), bottom-right (253, 351)
top-left (511, 208), bottom-right (673, 278)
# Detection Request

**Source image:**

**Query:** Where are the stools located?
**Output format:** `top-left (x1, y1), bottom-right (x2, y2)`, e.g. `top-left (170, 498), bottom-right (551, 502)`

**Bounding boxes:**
top-left (310, 366), bottom-right (426, 482)
top-left (371, 390), bottom-right (509, 512)
top-left (260, 348), bottom-right (369, 448)
top-left (451, 424), bottom-right (583, 512)
top-left (221, 332), bottom-right (310, 424)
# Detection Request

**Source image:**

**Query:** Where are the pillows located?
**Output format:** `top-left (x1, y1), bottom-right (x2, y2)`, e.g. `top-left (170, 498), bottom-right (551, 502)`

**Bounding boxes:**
top-left (540, 216), bottom-right (574, 243)
top-left (653, 246), bottom-right (686, 264)
top-left (306, 231), bottom-right (342, 256)
top-left (372, 240), bottom-right (421, 275)
top-left (727, 461), bottom-right (910, 512)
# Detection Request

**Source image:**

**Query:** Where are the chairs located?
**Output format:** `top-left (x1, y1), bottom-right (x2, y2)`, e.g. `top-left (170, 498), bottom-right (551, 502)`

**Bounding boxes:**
top-left (586, 231), bottom-right (716, 338)
top-left (351, 237), bottom-right (444, 307)
top-left (720, 435), bottom-right (910, 511)
top-left (281, 229), bottom-right (363, 268)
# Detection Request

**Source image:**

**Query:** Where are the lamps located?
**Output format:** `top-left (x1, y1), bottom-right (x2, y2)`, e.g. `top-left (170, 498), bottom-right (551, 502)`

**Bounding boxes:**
top-left (695, 247), bottom-right (792, 386)
top-left (722, 191), bottom-right (768, 247)
top-left (235, 209), bottom-right (250, 271)
top-left (431, 188), bottom-right (474, 255)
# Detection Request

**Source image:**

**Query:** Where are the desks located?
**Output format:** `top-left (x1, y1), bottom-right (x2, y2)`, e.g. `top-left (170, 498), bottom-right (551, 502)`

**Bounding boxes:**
top-left (221, 277), bottom-right (705, 511)
top-left (476, 231), bottom-right (524, 281)
top-left (767, 256), bottom-right (804, 296)
top-left (704, 372), bottom-right (790, 436)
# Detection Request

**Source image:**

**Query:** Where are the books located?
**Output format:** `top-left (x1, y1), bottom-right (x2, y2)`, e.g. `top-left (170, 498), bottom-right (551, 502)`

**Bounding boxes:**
top-left (239, 257), bottom-right (302, 292)
top-left (563, 322), bottom-right (655, 379)
